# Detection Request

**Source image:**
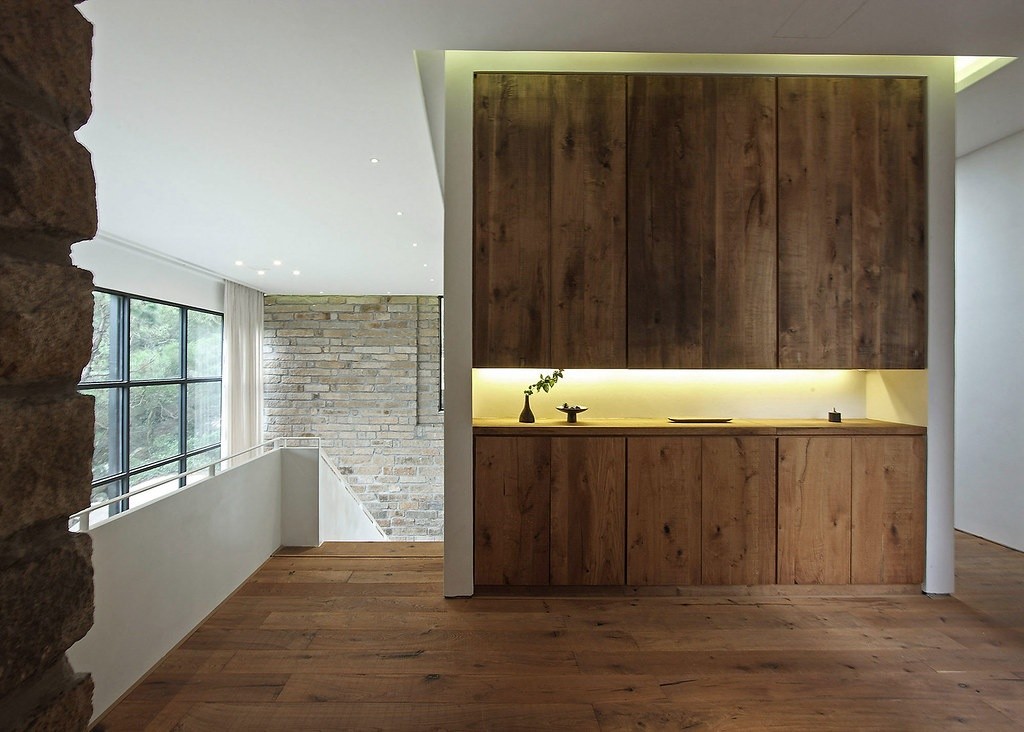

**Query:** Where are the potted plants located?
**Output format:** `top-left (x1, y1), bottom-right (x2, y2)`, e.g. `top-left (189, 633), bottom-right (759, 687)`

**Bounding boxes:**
top-left (518, 368), bottom-right (565, 423)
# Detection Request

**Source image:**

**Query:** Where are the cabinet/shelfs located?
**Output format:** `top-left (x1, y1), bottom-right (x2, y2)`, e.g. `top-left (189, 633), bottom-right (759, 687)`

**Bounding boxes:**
top-left (776, 435), bottom-right (926, 585)
top-left (475, 435), bottom-right (626, 586)
top-left (626, 436), bottom-right (777, 586)
top-left (626, 72), bottom-right (777, 369)
top-left (472, 72), bottom-right (627, 368)
top-left (777, 74), bottom-right (929, 369)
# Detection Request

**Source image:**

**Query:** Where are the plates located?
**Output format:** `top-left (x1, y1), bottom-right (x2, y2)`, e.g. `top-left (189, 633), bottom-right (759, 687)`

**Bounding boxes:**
top-left (668, 417), bottom-right (732, 423)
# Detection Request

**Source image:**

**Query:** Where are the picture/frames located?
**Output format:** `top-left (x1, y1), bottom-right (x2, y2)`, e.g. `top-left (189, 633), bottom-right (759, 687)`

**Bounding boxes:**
top-left (437, 296), bottom-right (445, 413)
